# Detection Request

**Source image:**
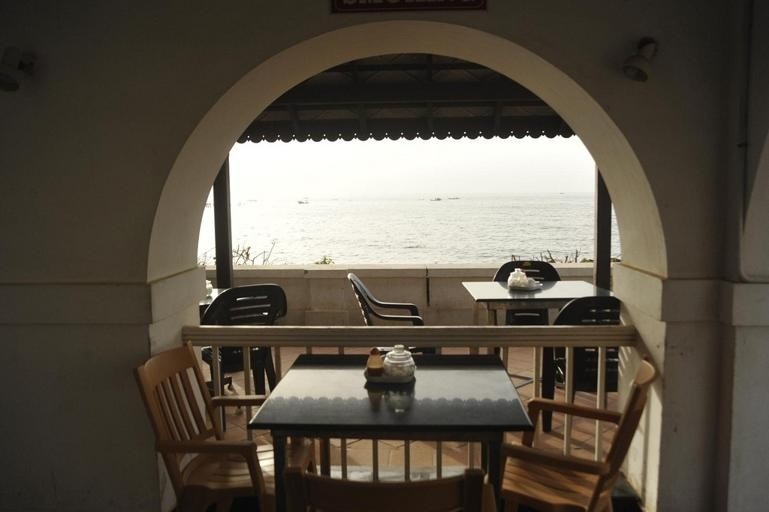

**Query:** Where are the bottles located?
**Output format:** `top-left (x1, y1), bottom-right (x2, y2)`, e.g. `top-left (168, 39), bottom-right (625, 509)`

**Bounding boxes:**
top-left (366, 347), bottom-right (383, 378)
top-left (368, 391), bottom-right (383, 412)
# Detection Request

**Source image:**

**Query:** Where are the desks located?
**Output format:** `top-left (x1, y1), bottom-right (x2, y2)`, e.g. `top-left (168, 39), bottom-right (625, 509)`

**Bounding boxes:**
top-left (457, 272), bottom-right (618, 355)
top-left (243, 349), bottom-right (537, 508)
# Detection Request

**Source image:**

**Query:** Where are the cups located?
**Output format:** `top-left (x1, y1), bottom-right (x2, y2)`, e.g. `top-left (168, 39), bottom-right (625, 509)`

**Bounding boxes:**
top-left (508, 268), bottom-right (528, 288)
top-left (381, 344), bottom-right (416, 385)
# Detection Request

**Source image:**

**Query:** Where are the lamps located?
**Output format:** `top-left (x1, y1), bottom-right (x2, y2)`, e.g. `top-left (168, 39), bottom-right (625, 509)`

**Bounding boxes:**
top-left (623, 38), bottom-right (663, 83)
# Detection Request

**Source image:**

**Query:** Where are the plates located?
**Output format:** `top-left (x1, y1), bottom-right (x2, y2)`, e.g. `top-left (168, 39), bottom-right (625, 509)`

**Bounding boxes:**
top-left (508, 283), bottom-right (544, 292)
top-left (363, 370), bottom-right (417, 384)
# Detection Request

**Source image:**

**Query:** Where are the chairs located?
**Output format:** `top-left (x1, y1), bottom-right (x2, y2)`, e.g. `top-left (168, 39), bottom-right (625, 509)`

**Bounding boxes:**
top-left (490, 376), bottom-right (655, 510)
top-left (134, 338), bottom-right (319, 509)
top-left (486, 261), bottom-right (566, 359)
top-left (196, 261), bottom-right (290, 433)
top-left (345, 271), bottom-right (443, 355)
top-left (539, 294), bottom-right (625, 434)
top-left (272, 463), bottom-right (490, 512)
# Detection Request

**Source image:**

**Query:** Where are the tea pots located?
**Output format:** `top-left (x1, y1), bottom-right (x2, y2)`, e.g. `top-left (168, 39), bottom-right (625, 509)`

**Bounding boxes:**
top-left (385, 390), bottom-right (415, 417)
top-left (204, 280), bottom-right (213, 297)
